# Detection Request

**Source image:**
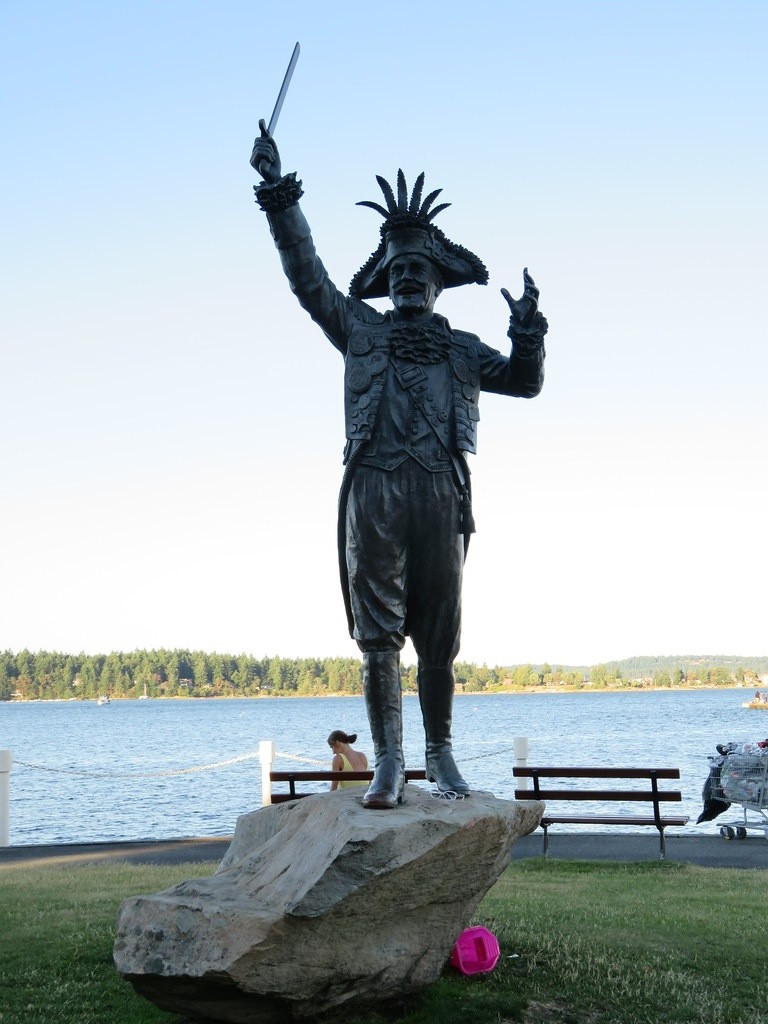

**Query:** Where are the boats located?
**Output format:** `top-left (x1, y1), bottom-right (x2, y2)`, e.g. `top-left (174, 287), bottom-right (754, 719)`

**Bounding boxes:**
top-left (748, 700), bottom-right (768, 710)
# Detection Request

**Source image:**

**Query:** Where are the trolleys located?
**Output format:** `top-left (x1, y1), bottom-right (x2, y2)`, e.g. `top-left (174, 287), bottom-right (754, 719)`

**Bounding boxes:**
top-left (706, 747), bottom-right (768, 841)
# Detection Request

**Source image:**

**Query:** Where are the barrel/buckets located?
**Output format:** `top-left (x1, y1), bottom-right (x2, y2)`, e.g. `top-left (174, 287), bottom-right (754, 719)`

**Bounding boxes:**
top-left (451, 925), bottom-right (500, 975)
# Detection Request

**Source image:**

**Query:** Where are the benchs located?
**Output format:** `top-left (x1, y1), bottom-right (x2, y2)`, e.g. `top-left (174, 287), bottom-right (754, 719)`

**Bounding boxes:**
top-left (512, 766), bottom-right (690, 862)
top-left (269, 769), bottom-right (426, 804)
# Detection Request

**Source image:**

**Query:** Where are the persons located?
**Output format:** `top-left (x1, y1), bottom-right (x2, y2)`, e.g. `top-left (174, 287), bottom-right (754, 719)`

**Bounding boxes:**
top-left (327, 730), bottom-right (371, 792)
top-left (252, 119), bottom-right (546, 811)
